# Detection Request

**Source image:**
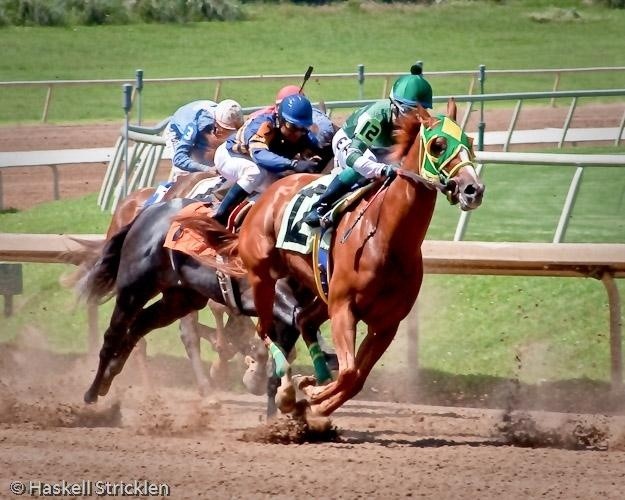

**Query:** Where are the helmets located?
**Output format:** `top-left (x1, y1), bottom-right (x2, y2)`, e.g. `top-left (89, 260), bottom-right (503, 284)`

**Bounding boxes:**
top-left (389, 75), bottom-right (432, 109)
top-left (215, 99), bottom-right (244, 130)
top-left (275, 85), bottom-right (313, 126)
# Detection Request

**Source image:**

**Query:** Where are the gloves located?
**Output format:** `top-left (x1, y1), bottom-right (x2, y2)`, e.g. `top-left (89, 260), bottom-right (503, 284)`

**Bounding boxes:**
top-left (295, 160), bottom-right (318, 173)
top-left (381, 166), bottom-right (398, 177)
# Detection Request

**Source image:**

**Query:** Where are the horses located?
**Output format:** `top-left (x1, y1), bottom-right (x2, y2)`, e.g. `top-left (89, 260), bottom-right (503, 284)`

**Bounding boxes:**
top-left (67, 93), bottom-right (487, 420)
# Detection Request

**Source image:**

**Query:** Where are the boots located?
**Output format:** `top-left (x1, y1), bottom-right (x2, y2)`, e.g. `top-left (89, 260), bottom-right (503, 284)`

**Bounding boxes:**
top-left (305, 174), bottom-right (351, 228)
top-left (211, 181), bottom-right (251, 229)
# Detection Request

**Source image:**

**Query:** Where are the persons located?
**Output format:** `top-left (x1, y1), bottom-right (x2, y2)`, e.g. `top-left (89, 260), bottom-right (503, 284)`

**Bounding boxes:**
top-left (210, 95), bottom-right (332, 226)
top-left (245, 84), bottom-right (339, 174)
top-left (143, 99), bottom-right (244, 208)
top-left (300, 66), bottom-right (432, 225)
